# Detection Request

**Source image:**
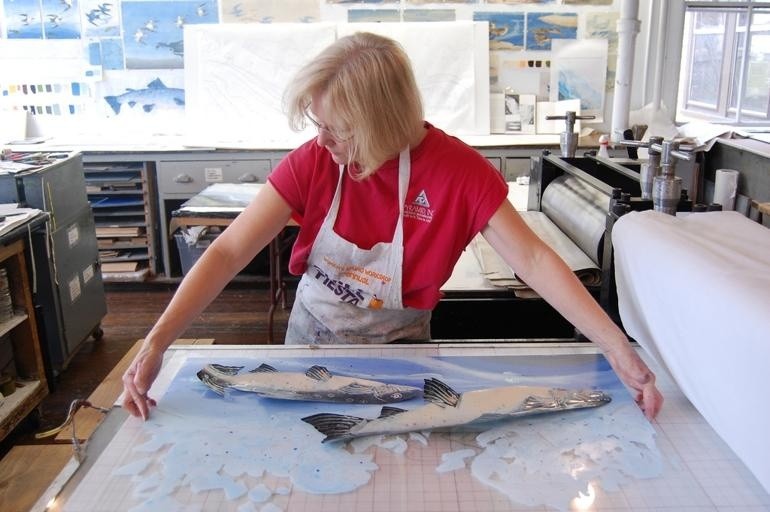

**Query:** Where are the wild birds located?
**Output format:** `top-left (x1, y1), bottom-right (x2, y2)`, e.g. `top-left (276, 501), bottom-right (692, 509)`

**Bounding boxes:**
top-left (47, 0), bottom-right (79, 28)
top-left (134, 1), bottom-right (208, 43)
top-left (19, 13), bottom-right (35, 27)
top-left (227, 2), bottom-right (313, 23)
top-left (85, 4), bottom-right (111, 28)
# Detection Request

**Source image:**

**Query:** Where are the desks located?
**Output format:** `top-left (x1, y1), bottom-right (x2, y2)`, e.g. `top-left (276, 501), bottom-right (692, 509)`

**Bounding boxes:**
top-left (171, 180), bottom-right (302, 344)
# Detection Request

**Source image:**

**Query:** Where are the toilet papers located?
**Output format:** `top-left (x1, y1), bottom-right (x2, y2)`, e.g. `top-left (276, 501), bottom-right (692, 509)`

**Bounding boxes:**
top-left (713, 167), bottom-right (738, 210)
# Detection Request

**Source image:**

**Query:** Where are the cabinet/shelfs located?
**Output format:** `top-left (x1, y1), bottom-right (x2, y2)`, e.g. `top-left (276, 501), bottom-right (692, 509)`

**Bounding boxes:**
top-left (0, 235), bottom-right (50, 444)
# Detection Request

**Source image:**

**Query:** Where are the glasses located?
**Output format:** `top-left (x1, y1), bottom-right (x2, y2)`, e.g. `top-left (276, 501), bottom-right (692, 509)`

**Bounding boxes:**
top-left (303, 102), bottom-right (354, 143)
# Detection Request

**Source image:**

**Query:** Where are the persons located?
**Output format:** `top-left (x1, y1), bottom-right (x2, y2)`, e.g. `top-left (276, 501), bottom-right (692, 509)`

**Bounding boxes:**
top-left (122, 30), bottom-right (666, 424)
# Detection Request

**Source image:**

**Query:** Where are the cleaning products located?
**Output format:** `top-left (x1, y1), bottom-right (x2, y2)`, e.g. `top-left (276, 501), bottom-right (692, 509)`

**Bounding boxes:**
top-left (597, 133), bottom-right (609, 157)
